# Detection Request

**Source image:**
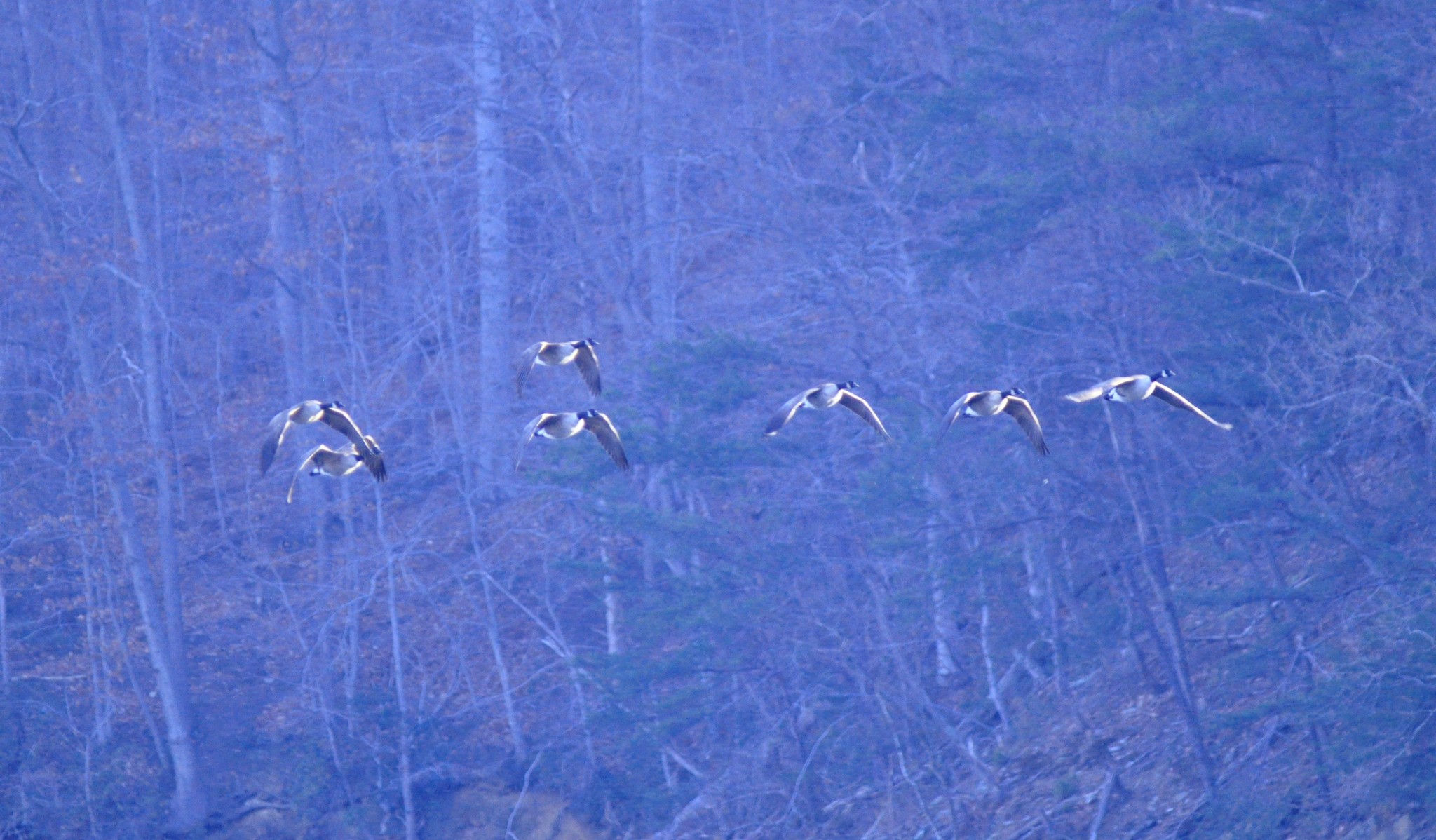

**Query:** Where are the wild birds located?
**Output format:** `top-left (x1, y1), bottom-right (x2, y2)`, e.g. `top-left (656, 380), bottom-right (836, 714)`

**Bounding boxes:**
top-left (759, 381), bottom-right (895, 445)
top-left (286, 435), bottom-right (389, 501)
top-left (515, 409), bottom-right (629, 475)
top-left (260, 401), bottom-right (381, 473)
top-left (932, 389), bottom-right (1048, 455)
top-left (1062, 370), bottom-right (1234, 430)
top-left (517, 339), bottom-right (604, 401)
